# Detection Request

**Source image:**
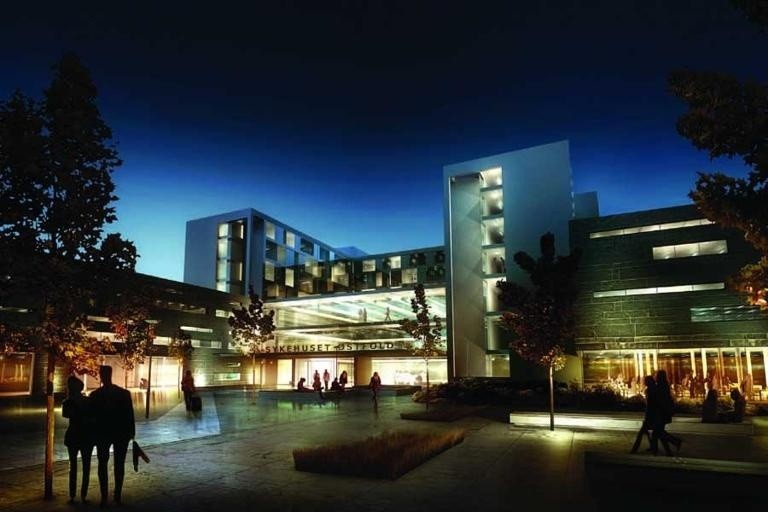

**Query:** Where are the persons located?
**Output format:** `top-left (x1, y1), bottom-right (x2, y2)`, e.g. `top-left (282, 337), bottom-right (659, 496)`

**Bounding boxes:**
top-left (313, 370), bottom-right (320, 378)
top-left (362, 308), bottom-right (368, 322)
top-left (384, 307), bottom-right (392, 321)
top-left (369, 371), bottom-right (381, 402)
top-left (313, 377), bottom-right (321, 391)
top-left (646, 369), bottom-right (674, 452)
top-left (682, 369), bottom-right (756, 424)
top-left (62, 374), bottom-right (93, 505)
top-left (332, 377), bottom-right (344, 392)
top-left (89, 364), bottom-right (136, 506)
top-left (631, 375), bottom-right (683, 453)
top-left (359, 309), bottom-right (363, 322)
top-left (323, 369), bottom-right (330, 390)
top-left (339, 370), bottom-right (347, 388)
top-left (181, 370), bottom-right (195, 410)
top-left (298, 378), bottom-right (314, 393)
top-left (140, 377), bottom-right (148, 389)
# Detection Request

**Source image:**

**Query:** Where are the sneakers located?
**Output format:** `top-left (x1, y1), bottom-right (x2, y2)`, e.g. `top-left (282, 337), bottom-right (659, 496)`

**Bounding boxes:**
top-left (67, 497), bottom-right (121, 510)
top-left (629, 438), bottom-right (682, 456)
